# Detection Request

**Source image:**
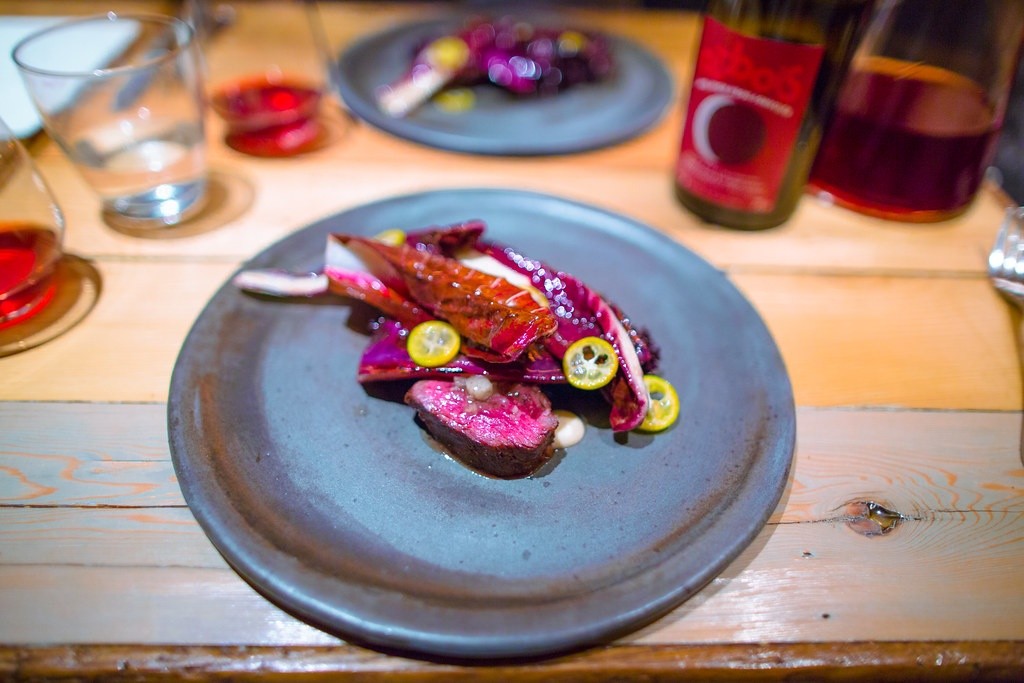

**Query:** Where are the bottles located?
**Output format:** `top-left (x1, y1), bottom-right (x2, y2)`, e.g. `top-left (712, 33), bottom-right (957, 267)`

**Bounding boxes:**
top-left (799, 0), bottom-right (1023, 229)
top-left (672, 0), bottom-right (863, 232)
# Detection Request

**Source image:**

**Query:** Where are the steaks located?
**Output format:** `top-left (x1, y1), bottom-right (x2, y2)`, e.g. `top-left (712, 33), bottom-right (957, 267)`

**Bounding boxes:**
top-left (402, 375), bottom-right (560, 479)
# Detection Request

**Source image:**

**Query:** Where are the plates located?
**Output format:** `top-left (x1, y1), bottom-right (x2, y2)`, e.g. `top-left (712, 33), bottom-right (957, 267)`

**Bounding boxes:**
top-left (167, 187), bottom-right (797, 658)
top-left (334, 16), bottom-right (672, 154)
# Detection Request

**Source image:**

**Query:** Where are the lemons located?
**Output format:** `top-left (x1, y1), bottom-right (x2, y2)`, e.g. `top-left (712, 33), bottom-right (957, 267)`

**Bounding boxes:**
top-left (406, 320), bottom-right (679, 432)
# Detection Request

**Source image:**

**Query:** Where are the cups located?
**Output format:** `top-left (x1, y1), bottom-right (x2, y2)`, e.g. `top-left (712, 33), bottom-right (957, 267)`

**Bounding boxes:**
top-left (178, 0), bottom-right (331, 156)
top-left (10, 12), bottom-right (213, 232)
top-left (0, 107), bottom-right (102, 357)
